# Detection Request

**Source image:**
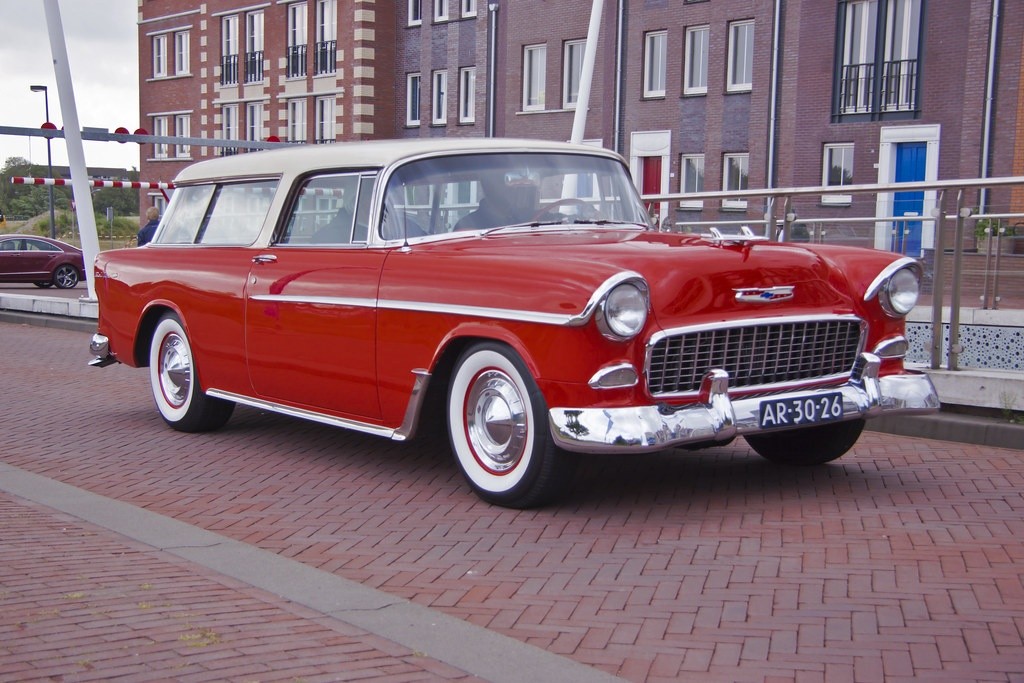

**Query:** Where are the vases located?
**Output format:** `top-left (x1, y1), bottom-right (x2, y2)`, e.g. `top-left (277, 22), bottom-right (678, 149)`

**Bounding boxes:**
top-left (976, 238), bottom-right (1016, 255)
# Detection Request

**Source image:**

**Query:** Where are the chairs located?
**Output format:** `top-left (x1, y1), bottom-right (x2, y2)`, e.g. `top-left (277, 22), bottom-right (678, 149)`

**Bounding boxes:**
top-left (453, 180), bottom-right (566, 232)
top-left (4, 242), bottom-right (15, 250)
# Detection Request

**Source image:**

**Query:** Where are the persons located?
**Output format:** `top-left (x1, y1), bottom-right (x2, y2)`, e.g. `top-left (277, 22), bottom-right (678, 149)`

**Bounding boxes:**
top-left (138, 207), bottom-right (161, 246)
top-left (311, 176), bottom-right (428, 243)
top-left (455, 172), bottom-right (567, 234)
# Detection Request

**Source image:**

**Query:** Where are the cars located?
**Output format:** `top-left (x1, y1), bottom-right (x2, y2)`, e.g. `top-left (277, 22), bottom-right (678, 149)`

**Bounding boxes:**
top-left (89, 137), bottom-right (945, 512)
top-left (0, 233), bottom-right (87, 289)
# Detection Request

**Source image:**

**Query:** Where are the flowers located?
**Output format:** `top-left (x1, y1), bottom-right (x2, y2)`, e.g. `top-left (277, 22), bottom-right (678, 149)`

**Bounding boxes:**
top-left (970, 207), bottom-right (1021, 237)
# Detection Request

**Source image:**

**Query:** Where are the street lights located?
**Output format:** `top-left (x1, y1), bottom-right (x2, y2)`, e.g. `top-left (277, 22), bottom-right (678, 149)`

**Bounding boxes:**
top-left (30, 85), bottom-right (57, 240)
